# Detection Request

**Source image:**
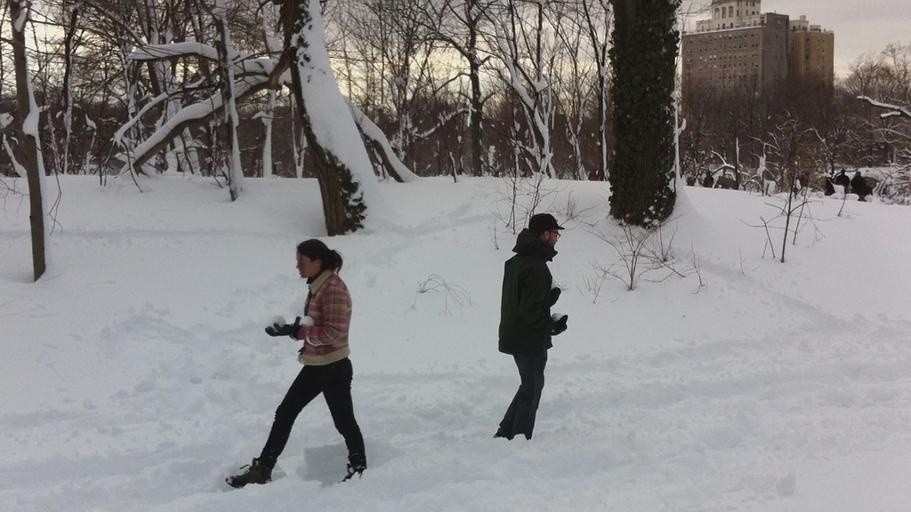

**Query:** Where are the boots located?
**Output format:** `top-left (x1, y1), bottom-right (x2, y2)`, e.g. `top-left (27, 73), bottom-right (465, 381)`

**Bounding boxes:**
top-left (343, 453), bottom-right (367, 482)
top-left (224, 458), bottom-right (275, 490)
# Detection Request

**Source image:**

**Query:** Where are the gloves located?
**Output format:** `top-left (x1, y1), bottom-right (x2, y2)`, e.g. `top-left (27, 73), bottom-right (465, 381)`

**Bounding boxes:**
top-left (289, 316), bottom-right (302, 339)
top-left (549, 287), bottom-right (561, 306)
top-left (265, 322), bottom-right (292, 340)
top-left (550, 314), bottom-right (568, 336)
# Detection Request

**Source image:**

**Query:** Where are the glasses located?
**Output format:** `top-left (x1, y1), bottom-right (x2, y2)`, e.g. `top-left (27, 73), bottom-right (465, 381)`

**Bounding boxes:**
top-left (553, 232), bottom-right (561, 240)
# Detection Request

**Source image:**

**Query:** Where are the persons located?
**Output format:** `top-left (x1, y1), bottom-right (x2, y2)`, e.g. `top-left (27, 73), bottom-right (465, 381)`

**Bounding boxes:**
top-left (587, 167), bottom-right (605, 181)
top-left (685, 165), bottom-right (868, 202)
top-left (224, 238), bottom-right (367, 489)
top-left (491, 213), bottom-right (569, 440)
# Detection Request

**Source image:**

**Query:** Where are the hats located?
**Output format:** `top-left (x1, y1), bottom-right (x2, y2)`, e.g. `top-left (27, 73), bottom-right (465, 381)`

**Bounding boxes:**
top-left (528, 213), bottom-right (565, 234)
